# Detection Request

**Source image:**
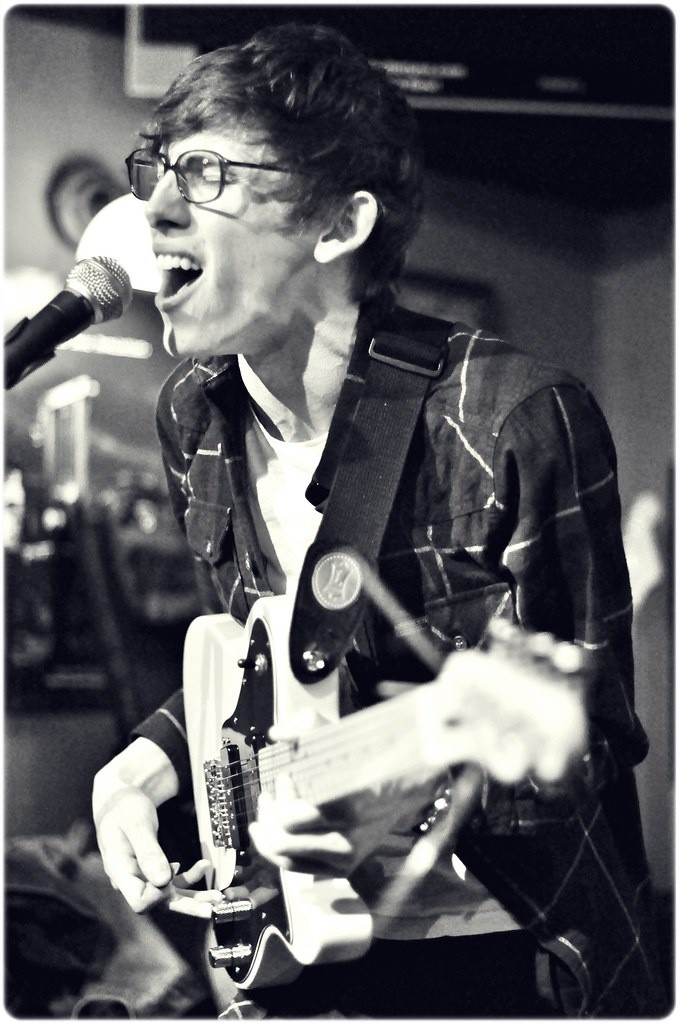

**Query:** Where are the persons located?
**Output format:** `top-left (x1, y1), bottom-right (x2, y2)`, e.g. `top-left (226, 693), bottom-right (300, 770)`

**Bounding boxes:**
top-left (93, 26), bottom-right (663, 1020)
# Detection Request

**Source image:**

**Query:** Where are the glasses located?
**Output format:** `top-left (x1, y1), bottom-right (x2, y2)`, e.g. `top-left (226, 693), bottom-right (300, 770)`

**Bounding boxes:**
top-left (125, 147), bottom-right (389, 219)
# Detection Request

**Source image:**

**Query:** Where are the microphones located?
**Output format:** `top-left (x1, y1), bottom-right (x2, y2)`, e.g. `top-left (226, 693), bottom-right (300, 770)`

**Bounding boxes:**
top-left (4, 256), bottom-right (133, 391)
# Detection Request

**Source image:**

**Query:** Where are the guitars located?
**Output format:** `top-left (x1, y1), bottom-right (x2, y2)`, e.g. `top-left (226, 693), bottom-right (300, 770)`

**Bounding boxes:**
top-left (178, 579), bottom-right (610, 994)
